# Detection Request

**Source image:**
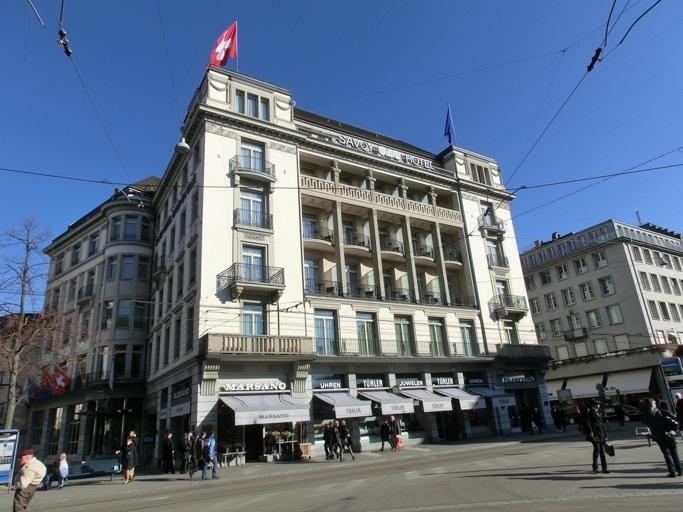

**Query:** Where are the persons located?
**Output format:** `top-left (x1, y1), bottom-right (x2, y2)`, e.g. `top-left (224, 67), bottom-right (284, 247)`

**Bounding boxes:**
top-left (520, 403), bottom-right (567, 434)
top-left (180, 431), bottom-right (220, 481)
top-left (121, 436), bottom-right (138, 484)
top-left (613, 401), bottom-right (626, 426)
top-left (585, 400), bottom-right (610, 473)
top-left (12, 448), bottom-right (48, 512)
top-left (323, 419), bottom-right (355, 462)
top-left (389, 416), bottom-right (401, 451)
top-left (54, 453), bottom-right (70, 490)
top-left (379, 418), bottom-right (393, 451)
top-left (163, 432), bottom-right (176, 474)
top-left (638, 392), bottom-right (683, 478)
top-left (129, 431), bottom-right (139, 476)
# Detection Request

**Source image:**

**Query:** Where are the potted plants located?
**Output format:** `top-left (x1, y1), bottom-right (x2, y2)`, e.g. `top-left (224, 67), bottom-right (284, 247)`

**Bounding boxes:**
top-left (263, 434), bottom-right (275, 462)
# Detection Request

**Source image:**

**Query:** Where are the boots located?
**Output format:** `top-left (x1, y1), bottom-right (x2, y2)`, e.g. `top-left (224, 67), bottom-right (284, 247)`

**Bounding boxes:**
top-left (592, 463), bottom-right (610, 473)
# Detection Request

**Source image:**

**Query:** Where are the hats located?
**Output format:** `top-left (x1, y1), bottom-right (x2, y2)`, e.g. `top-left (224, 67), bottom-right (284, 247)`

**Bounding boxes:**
top-left (19, 448), bottom-right (36, 456)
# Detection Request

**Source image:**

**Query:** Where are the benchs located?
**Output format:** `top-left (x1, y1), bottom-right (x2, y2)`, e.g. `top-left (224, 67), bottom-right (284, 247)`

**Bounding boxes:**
top-left (43, 458), bottom-right (123, 491)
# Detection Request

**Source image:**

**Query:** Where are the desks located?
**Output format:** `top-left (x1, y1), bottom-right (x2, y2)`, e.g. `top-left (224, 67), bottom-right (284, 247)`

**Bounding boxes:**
top-left (276, 440), bottom-right (298, 460)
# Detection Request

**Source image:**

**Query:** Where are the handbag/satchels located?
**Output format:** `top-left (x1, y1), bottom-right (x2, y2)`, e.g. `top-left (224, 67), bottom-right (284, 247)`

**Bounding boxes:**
top-left (605, 444), bottom-right (615, 456)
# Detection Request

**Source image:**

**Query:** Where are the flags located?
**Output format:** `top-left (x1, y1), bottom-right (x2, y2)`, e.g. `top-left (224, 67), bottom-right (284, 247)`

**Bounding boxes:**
top-left (204, 21), bottom-right (237, 69)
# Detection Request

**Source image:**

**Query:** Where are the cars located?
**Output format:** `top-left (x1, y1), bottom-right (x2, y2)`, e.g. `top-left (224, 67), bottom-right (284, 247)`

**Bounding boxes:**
top-left (563, 404), bottom-right (640, 425)
